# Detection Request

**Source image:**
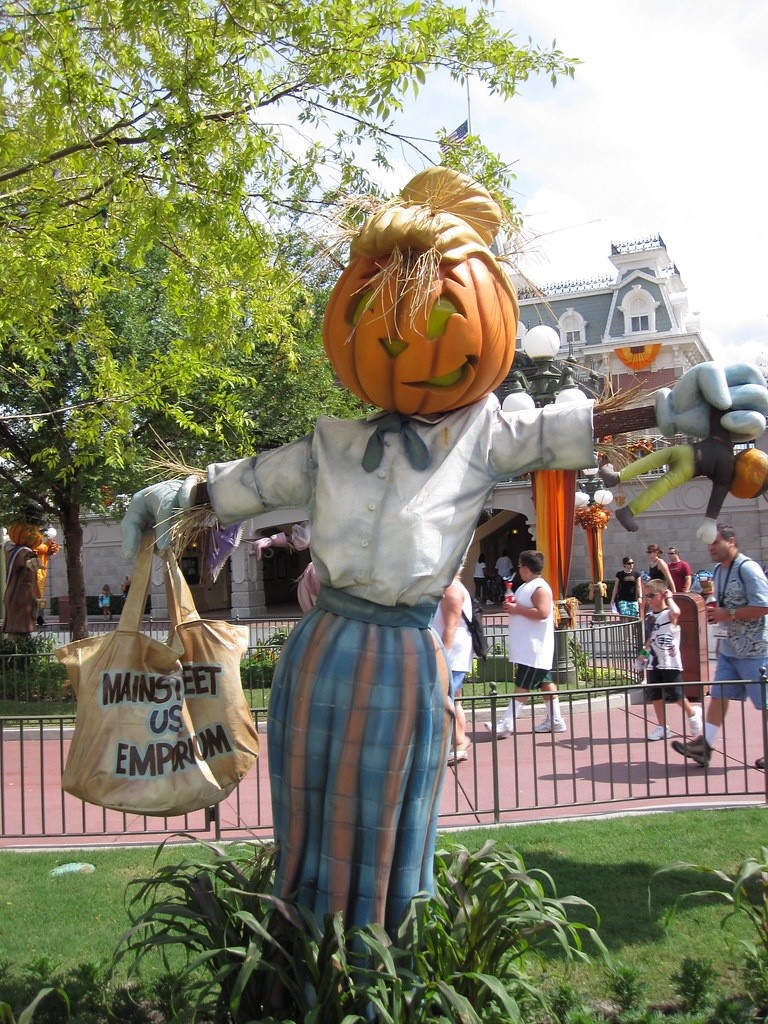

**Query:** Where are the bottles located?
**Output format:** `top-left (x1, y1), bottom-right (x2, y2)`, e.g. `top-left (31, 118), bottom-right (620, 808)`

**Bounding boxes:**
top-left (504, 583), bottom-right (516, 604)
top-left (705, 592), bottom-right (718, 625)
top-left (642, 572), bottom-right (650, 583)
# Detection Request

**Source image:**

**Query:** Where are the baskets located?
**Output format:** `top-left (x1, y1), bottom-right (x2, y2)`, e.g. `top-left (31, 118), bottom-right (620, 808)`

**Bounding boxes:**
top-left (627, 659), bottom-right (676, 704)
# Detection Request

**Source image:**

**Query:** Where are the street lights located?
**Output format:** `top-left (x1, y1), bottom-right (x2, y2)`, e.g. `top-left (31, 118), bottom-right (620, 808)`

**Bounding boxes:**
top-left (494, 323), bottom-right (603, 684)
top-left (574, 464), bottom-right (615, 622)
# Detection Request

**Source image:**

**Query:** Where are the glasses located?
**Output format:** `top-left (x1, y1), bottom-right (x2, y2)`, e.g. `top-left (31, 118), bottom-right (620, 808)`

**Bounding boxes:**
top-left (644, 592), bottom-right (662, 598)
top-left (646, 550), bottom-right (655, 553)
top-left (668, 553), bottom-right (676, 554)
top-left (624, 562), bottom-right (633, 565)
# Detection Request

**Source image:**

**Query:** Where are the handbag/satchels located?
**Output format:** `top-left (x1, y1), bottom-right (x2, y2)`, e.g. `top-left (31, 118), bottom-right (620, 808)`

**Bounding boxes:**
top-left (55, 522), bottom-right (264, 817)
top-left (610, 602), bottom-right (618, 614)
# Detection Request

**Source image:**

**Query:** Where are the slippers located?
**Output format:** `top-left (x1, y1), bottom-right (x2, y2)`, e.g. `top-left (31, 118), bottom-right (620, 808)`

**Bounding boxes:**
top-left (445, 742), bottom-right (473, 766)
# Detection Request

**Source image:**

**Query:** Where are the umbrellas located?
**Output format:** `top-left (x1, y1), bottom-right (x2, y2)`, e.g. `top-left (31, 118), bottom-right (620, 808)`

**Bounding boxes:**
top-left (209, 520), bottom-right (275, 583)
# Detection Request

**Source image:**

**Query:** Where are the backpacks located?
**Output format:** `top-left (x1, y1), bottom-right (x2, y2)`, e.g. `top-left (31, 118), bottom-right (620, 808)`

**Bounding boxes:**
top-left (461, 595), bottom-right (488, 663)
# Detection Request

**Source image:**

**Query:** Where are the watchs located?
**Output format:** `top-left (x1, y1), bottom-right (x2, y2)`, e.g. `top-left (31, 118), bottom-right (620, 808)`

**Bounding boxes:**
top-left (728, 608), bottom-right (736, 620)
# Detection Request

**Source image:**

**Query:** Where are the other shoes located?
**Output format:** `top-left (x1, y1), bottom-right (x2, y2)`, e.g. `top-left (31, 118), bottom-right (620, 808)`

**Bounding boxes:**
top-left (486, 599), bottom-right (495, 606)
top-left (475, 601), bottom-right (482, 608)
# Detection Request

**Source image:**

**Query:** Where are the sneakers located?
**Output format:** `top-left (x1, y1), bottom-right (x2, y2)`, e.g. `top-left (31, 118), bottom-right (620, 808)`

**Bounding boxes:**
top-left (647, 725), bottom-right (672, 741)
top-left (484, 717), bottom-right (513, 739)
top-left (672, 735), bottom-right (714, 767)
top-left (755, 757), bottom-right (764, 769)
top-left (687, 705), bottom-right (703, 736)
top-left (531, 714), bottom-right (567, 733)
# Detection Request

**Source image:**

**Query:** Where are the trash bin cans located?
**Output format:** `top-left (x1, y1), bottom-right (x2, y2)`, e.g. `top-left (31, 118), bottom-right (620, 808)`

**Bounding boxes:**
top-left (58, 595), bottom-right (71, 630)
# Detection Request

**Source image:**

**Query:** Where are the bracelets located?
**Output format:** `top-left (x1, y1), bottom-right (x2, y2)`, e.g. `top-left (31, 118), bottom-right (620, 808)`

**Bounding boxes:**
top-left (637, 596), bottom-right (642, 598)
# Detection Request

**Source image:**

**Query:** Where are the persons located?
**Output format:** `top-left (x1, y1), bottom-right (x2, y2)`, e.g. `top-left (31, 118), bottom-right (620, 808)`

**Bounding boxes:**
top-left (609, 556), bottom-right (642, 616)
top-left (637, 579), bottom-right (704, 741)
top-left (297, 562), bottom-right (320, 614)
top-left (645, 543), bottom-right (677, 594)
top-left (667, 547), bottom-right (693, 592)
top-left (670, 522), bottom-right (768, 768)
top-left (2, 502), bottom-right (50, 640)
top-left (495, 551), bottom-right (515, 602)
top-left (120, 575), bottom-right (130, 599)
top-left (251, 521), bottom-right (310, 561)
top-left (430, 557), bottom-right (475, 767)
top-left (483, 548), bottom-right (567, 740)
top-left (119, 166), bottom-right (768, 960)
top-left (99, 584), bottom-right (113, 621)
top-left (597, 394), bottom-right (768, 545)
top-left (473, 553), bottom-right (496, 605)
